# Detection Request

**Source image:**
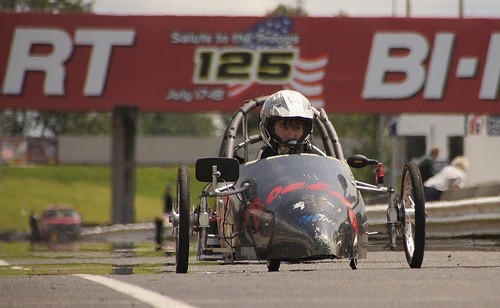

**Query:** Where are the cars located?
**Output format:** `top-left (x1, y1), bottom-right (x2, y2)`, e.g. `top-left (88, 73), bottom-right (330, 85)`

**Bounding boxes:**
top-left (35, 205), bottom-right (81, 240)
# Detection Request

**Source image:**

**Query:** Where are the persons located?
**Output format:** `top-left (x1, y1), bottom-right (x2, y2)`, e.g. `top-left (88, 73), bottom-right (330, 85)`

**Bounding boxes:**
top-left (255, 89), bottom-right (328, 160)
top-left (418, 150), bottom-right (440, 182)
top-left (423, 157), bottom-right (468, 201)
top-left (30, 209), bottom-right (42, 253)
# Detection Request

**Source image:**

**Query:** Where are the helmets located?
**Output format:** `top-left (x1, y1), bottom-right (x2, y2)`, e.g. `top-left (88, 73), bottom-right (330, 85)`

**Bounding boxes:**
top-left (258, 89), bottom-right (314, 154)
